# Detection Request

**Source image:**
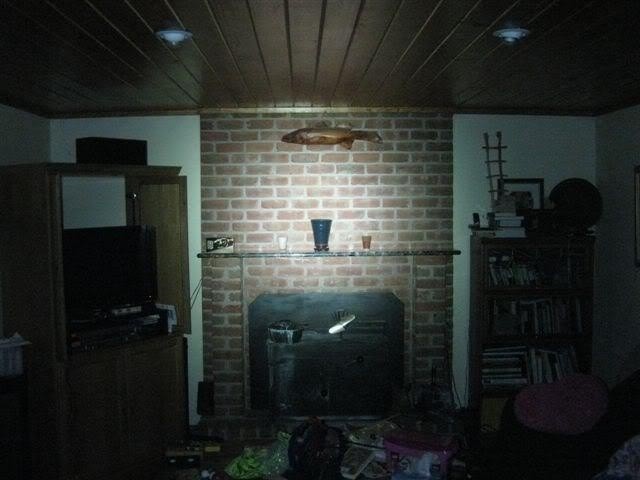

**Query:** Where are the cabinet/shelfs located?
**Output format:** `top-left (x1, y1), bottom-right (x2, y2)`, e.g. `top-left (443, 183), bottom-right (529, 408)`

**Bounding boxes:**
top-left (466, 239), bottom-right (597, 430)
top-left (0, 163), bottom-right (192, 480)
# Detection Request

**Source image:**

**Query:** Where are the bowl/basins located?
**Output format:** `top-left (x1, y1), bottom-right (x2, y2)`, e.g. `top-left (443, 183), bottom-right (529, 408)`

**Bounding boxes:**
top-left (267, 326), bottom-right (303, 345)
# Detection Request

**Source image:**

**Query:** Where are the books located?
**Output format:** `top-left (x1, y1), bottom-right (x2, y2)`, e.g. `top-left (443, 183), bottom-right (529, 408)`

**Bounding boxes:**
top-left (480, 250), bottom-right (587, 395)
top-left (470, 194), bottom-right (528, 238)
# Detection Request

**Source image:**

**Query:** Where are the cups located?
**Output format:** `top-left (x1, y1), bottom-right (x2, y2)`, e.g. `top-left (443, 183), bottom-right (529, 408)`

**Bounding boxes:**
top-left (311, 219), bottom-right (332, 252)
top-left (362, 236), bottom-right (371, 249)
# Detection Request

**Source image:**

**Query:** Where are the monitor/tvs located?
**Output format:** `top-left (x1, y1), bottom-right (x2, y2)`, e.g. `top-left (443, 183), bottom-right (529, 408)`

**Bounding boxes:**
top-left (62, 224), bottom-right (159, 320)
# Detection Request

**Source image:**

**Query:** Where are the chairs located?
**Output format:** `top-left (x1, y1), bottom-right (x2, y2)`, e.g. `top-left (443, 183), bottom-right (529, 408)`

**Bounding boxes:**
top-left (494, 371), bottom-right (638, 480)
